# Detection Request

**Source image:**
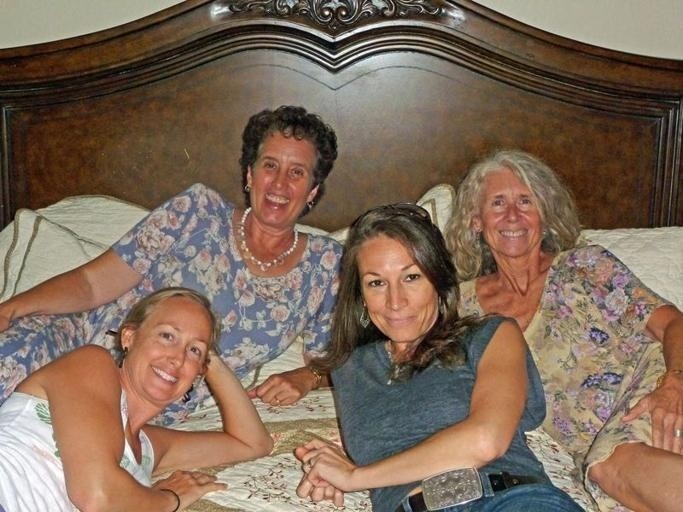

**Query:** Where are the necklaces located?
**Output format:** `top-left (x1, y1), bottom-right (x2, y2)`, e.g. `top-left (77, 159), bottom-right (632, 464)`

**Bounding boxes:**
top-left (384, 338), bottom-right (424, 386)
top-left (239, 205), bottom-right (299, 270)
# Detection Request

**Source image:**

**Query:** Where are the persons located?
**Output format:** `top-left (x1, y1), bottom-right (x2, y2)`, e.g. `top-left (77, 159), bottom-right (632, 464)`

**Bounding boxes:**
top-left (448, 149), bottom-right (682, 511)
top-left (294, 203), bottom-right (583, 510)
top-left (0, 104), bottom-right (345, 407)
top-left (1, 287), bottom-right (273, 511)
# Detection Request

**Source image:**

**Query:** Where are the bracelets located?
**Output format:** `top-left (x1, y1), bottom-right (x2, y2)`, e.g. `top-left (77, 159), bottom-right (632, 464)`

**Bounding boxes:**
top-left (160, 489), bottom-right (180, 509)
top-left (304, 363), bottom-right (326, 386)
top-left (656, 368), bottom-right (680, 385)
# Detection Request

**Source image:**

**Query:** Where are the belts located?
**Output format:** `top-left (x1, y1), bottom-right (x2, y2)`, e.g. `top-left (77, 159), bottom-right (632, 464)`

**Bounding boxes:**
top-left (396, 465), bottom-right (540, 511)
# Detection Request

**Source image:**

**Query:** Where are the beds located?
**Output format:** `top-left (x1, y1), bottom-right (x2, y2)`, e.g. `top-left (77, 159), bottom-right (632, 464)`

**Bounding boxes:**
top-left (0, 0), bottom-right (683, 512)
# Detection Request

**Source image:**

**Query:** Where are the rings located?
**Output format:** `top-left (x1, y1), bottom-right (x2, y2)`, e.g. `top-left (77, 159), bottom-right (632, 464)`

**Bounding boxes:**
top-left (671, 427), bottom-right (680, 437)
top-left (273, 396), bottom-right (281, 404)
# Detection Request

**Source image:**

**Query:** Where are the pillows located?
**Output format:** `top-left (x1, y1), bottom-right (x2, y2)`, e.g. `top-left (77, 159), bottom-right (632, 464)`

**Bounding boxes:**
top-left (294, 179), bottom-right (459, 248)
top-left (0, 206), bottom-right (116, 300)
top-left (583, 221), bottom-right (683, 310)
